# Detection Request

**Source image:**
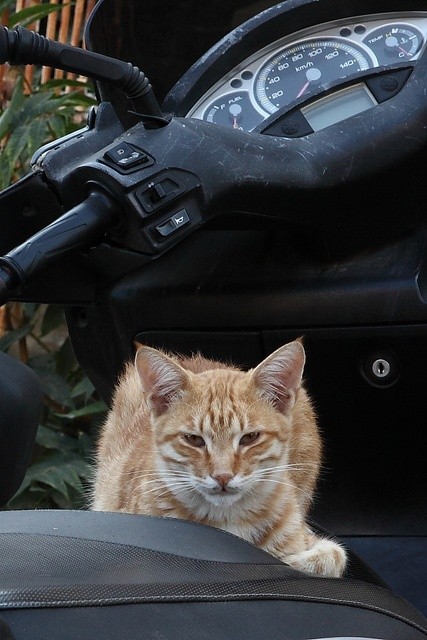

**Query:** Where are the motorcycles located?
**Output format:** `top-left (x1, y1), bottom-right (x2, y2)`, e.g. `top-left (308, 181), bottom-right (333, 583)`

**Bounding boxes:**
top-left (2, 1), bottom-right (427, 638)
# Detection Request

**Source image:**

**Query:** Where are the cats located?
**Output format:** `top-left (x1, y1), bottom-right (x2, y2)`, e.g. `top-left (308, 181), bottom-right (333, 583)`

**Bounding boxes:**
top-left (82, 338), bottom-right (348, 579)
